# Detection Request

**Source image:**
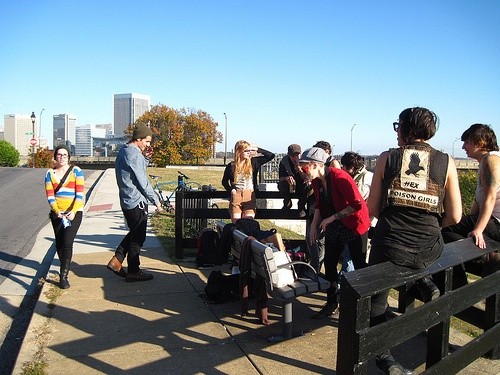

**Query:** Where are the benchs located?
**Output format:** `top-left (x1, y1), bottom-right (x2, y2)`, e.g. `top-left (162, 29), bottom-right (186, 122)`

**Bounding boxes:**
top-left (215, 220), bottom-right (330, 342)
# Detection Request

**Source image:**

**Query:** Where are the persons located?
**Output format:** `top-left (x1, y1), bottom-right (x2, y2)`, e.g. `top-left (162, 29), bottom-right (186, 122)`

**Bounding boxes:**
top-left (278, 143), bottom-right (311, 217)
top-left (45, 144), bottom-right (85, 289)
top-left (221, 141), bottom-right (275, 267)
top-left (440, 124), bottom-right (500, 289)
top-left (298, 148), bottom-right (371, 321)
top-left (305, 140), bottom-right (341, 263)
top-left (339, 150), bottom-right (372, 284)
top-left (107, 123), bottom-right (161, 282)
top-left (366, 105), bottom-right (464, 375)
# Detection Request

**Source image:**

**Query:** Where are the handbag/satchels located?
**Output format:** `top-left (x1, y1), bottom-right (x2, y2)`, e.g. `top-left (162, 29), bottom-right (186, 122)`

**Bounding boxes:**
top-left (271, 250), bottom-right (318, 290)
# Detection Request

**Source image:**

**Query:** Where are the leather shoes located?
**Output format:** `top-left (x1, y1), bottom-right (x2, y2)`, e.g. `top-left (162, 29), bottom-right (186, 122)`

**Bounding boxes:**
top-left (125, 270), bottom-right (153, 282)
top-left (107, 256), bottom-right (127, 278)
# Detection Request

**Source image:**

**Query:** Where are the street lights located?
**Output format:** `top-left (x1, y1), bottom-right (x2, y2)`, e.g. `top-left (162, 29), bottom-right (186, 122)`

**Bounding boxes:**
top-left (30, 111), bottom-right (37, 168)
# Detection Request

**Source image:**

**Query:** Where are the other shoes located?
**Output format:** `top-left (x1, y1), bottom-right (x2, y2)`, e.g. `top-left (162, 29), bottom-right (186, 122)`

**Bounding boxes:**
top-left (425, 284), bottom-right (440, 302)
top-left (317, 306), bottom-right (338, 317)
top-left (484, 252), bottom-right (500, 274)
top-left (383, 360), bottom-right (417, 375)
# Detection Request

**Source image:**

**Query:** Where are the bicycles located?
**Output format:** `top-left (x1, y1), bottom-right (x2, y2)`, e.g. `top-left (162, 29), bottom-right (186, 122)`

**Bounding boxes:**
top-left (146, 171), bottom-right (202, 218)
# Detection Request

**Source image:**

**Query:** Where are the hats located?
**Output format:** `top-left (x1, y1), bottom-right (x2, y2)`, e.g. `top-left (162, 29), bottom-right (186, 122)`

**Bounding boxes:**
top-left (299, 147), bottom-right (326, 164)
top-left (288, 144), bottom-right (302, 154)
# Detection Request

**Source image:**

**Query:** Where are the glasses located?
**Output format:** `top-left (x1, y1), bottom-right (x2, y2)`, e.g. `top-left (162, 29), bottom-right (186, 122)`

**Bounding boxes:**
top-left (57, 154), bottom-right (69, 158)
top-left (393, 122), bottom-right (402, 132)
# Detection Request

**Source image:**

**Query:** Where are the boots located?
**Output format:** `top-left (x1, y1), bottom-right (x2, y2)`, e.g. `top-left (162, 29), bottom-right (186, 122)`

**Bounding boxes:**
top-left (59, 258), bottom-right (71, 289)
